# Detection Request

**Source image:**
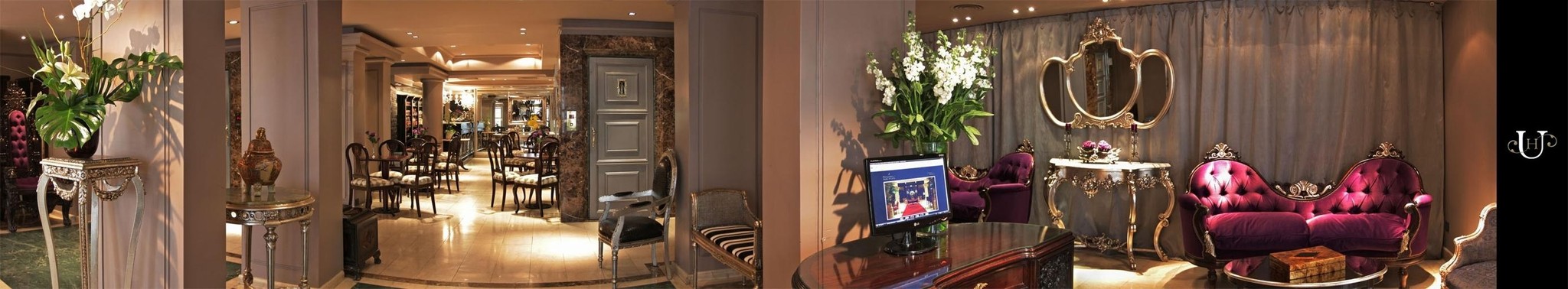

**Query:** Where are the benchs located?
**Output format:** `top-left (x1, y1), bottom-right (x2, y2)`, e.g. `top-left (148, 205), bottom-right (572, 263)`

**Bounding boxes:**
top-left (691, 189), bottom-right (763, 288)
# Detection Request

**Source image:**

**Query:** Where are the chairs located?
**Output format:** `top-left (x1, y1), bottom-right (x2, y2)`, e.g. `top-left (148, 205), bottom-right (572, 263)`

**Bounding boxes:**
top-left (947, 138), bottom-right (1036, 224)
top-left (597, 149), bottom-right (678, 289)
top-left (487, 131), bottom-right (559, 217)
top-left (346, 132), bottom-right (468, 217)
top-left (0, 81), bottom-right (73, 232)
top-left (1439, 203), bottom-right (1497, 289)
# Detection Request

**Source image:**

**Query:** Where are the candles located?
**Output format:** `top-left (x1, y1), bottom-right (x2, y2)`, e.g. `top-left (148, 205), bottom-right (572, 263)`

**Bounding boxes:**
top-left (1131, 124), bottom-right (1137, 132)
top-left (1066, 123), bottom-right (1071, 130)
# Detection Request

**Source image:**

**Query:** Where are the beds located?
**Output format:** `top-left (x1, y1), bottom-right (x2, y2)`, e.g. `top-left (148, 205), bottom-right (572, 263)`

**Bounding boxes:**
top-left (225, 187), bottom-right (315, 289)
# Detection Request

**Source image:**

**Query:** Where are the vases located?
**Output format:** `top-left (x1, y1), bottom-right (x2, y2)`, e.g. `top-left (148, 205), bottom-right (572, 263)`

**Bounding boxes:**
top-left (911, 142), bottom-right (949, 233)
top-left (238, 127), bottom-right (282, 196)
top-left (64, 118), bottom-right (99, 158)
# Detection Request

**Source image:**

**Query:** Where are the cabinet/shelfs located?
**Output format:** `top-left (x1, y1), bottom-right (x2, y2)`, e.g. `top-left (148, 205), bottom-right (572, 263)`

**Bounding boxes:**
top-left (791, 222), bottom-right (1075, 289)
top-left (397, 94), bottom-right (423, 149)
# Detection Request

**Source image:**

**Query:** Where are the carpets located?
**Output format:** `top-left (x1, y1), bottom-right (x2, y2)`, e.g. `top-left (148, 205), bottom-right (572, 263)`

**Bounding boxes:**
top-left (0, 222), bottom-right (241, 289)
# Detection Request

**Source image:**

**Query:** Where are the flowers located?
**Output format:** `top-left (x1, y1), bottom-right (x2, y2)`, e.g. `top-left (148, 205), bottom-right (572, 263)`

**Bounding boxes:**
top-left (1097, 140), bottom-right (1111, 152)
top-left (442, 113), bottom-right (458, 133)
top-left (1083, 140), bottom-right (1096, 151)
top-left (0, 0), bottom-right (183, 149)
top-left (865, 10), bottom-right (997, 149)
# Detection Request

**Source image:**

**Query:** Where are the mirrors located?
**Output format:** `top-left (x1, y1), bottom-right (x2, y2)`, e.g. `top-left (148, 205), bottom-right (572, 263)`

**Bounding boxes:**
top-left (1038, 17), bottom-right (1175, 130)
top-left (507, 96), bottom-right (546, 126)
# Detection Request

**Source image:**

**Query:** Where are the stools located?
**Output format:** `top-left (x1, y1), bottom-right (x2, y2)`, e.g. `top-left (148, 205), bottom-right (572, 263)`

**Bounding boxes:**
top-left (36, 157), bottom-right (147, 289)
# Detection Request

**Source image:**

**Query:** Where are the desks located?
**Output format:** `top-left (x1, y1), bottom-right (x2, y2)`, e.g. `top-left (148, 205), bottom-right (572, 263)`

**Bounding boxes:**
top-left (1043, 158), bottom-right (1176, 270)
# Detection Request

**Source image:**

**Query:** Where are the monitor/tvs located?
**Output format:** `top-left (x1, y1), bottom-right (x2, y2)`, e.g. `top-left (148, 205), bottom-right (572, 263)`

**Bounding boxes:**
top-left (863, 153), bottom-right (952, 255)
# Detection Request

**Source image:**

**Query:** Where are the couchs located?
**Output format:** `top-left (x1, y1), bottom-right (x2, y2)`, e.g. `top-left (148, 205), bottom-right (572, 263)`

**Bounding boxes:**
top-left (1178, 141), bottom-right (1433, 288)
